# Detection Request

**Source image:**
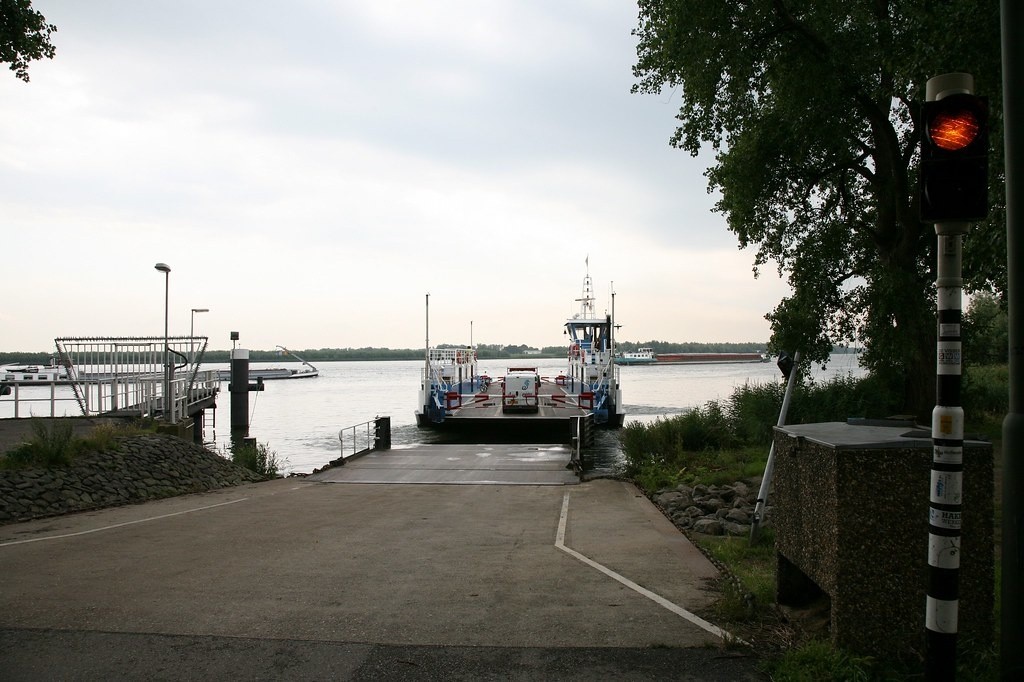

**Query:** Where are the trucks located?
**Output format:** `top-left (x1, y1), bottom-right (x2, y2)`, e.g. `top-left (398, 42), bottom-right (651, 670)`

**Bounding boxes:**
top-left (501, 366), bottom-right (541, 413)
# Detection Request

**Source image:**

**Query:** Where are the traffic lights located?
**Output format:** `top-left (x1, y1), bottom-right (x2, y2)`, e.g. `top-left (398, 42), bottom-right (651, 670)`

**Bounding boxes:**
top-left (916, 95), bottom-right (990, 226)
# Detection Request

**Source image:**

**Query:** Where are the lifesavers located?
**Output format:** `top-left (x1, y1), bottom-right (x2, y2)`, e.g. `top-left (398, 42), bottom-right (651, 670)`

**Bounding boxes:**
top-left (456, 351), bottom-right (462, 364)
top-left (574, 344), bottom-right (579, 357)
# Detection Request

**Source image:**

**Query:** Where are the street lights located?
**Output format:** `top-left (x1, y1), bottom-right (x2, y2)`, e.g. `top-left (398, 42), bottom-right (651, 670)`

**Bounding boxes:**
top-left (155, 263), bottom-right (171, 422)
top-left (188, 309), bottom-right (208, 371)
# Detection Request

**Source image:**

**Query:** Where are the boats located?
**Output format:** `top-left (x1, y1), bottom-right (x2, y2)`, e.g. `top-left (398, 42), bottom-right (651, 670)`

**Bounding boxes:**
top-left (613, 347), bottom-right (659, 365)
top-left (6, 364), bottom-right (39, 373)
top-left (43, 359), bottom-right (59, 369)
top-left (412, 254), bottom-right (627, 442)
top-left (276, 346), bottom-right (318, 378)
